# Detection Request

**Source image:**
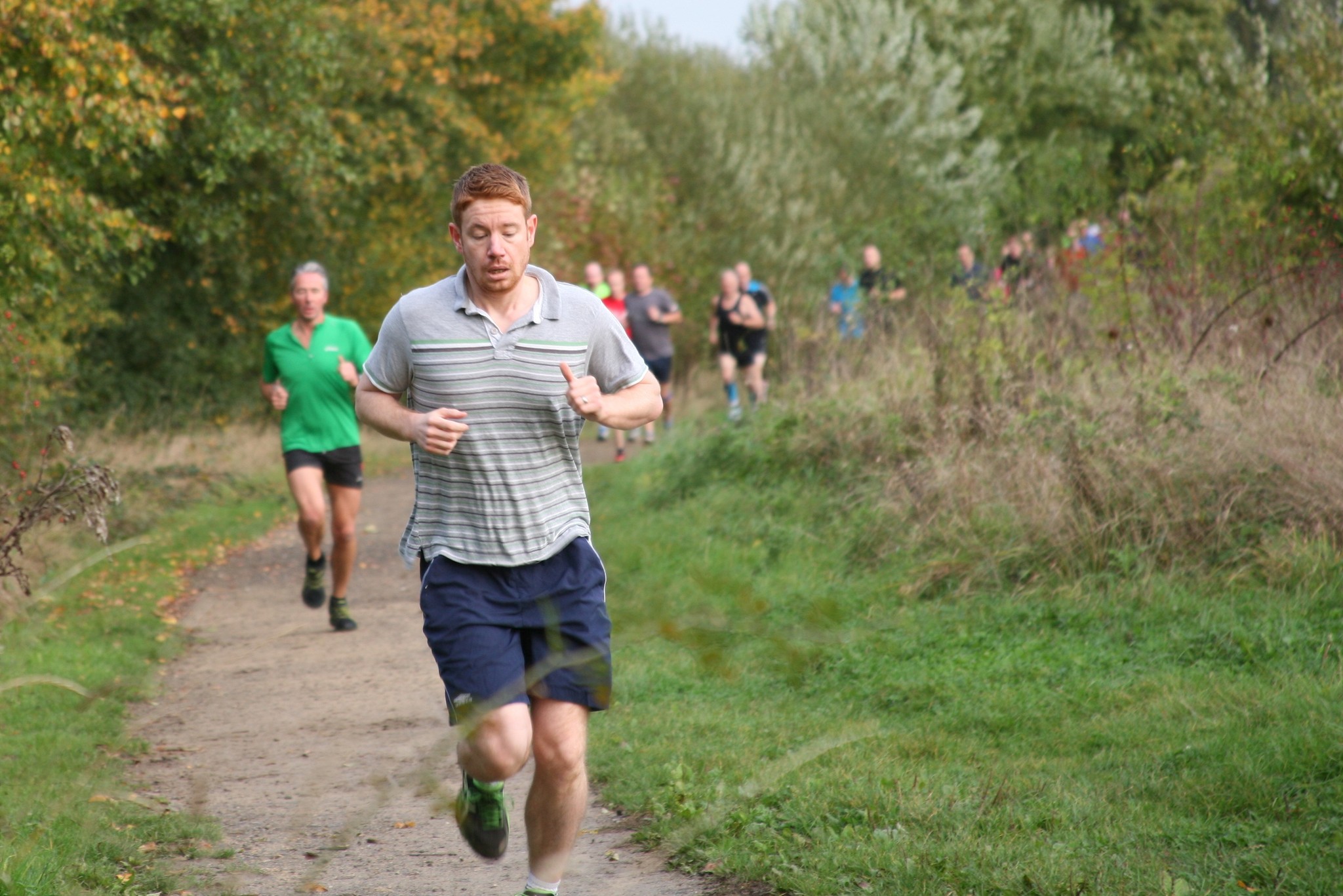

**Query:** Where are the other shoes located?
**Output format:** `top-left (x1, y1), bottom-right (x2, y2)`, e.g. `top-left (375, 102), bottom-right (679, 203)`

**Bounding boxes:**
top-left (615, 448), bottom-right (628, 462)
top-left (664, 415), bottom-right (673, 432)
top-left (730, 399), bottom-right (740, 421)
top-left (598, 429), bottom-right (609, 441)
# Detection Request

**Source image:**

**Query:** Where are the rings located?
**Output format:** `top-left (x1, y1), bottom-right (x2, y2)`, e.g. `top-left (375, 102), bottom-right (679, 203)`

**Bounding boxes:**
top-left (582, 395), bottom-right (588, 404)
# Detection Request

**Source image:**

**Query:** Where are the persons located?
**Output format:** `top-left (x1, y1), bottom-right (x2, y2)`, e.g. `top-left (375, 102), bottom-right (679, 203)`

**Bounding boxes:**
top-left (355, 163), bottom-right (664, 896)
top-left (951, 212), bottom-right (1141, 313)
top-left (261, 260), bottom-right (373, 631)
top-left (829, 243), bottom-right (909, 338)
top-left (576, 265), bottom-right (682, 463)
top-left (710, 258), bottom-right (777, 419)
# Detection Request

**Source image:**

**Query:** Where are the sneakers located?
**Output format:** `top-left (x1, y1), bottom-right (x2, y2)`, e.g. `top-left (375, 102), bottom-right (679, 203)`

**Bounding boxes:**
top-left (456, 763), bottom-right (510, 860)
top-left (301, 553), bottom-right (326, 608)
top-left (328, 598), bottom-right (357, 631)
